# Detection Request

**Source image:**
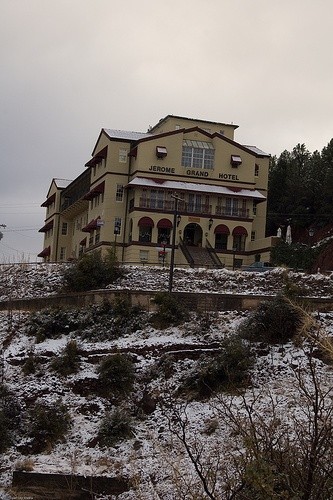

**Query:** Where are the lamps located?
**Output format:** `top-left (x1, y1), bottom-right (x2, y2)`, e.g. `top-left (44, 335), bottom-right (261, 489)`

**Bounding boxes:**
top-left (177, 215), bottom-right (182, 227)
top-left (205, 232), bottom-right (208, 237)
top-left (208, 218), bottom-right (213, 231)
top-left (179, 230), bottom-right (182, 235)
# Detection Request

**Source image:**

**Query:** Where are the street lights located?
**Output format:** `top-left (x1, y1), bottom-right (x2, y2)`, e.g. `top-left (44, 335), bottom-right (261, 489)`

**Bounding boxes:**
top-left (169, 193), bottom-right (185, 291)
